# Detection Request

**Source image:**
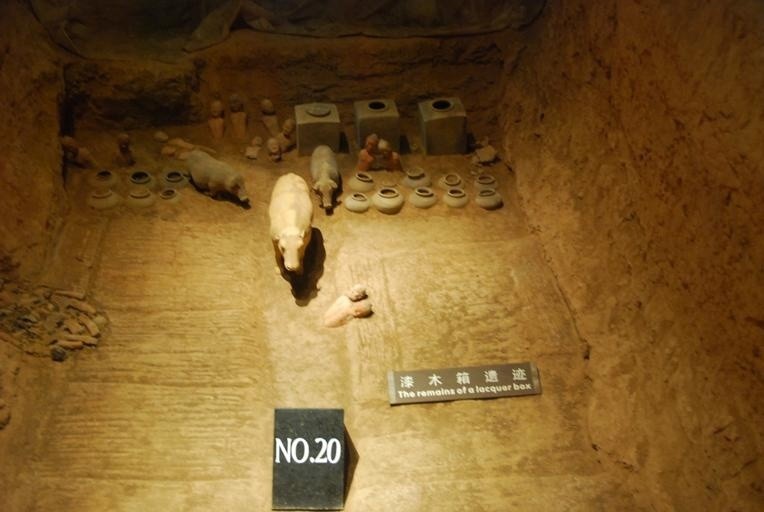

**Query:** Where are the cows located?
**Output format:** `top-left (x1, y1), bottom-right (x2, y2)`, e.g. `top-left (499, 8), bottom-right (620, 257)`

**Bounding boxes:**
top-left (268, 171), bottom-right (315, 272)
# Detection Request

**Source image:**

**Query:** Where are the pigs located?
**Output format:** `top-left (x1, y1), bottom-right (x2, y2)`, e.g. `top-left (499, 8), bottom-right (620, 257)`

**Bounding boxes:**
top-left (309, 143), bottom-right (340, 210)
top-left (185, 150), bottom-right (249, 202)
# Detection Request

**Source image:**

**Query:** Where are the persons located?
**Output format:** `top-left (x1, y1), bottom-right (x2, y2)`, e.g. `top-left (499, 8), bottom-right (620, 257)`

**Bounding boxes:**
top-left (63, 136), bottom-right (99, 170)
top-left (321, 298), bottom-right (373, 328)
top-left (206, 93), bottom-right (402, 174)
top-left (113, 132), bottom-right (134, 168)
top-left (323, 281), bottom-right (366, 320)
